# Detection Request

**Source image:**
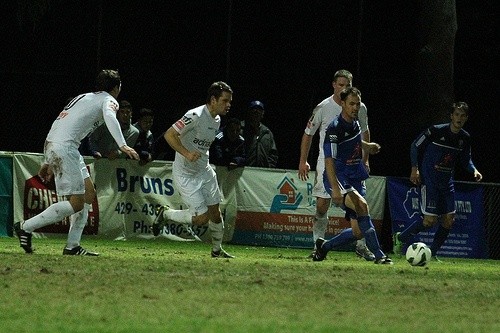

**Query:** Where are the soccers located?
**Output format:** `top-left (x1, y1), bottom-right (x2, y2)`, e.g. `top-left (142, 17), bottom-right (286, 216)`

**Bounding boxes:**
top-left (406, 242), bottom-right (432, 267)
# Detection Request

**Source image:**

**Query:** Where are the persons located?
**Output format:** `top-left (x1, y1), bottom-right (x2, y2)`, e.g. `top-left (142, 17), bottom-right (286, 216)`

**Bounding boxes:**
top-left (11, 67), bottom-right (139, 258)
top-left (391, 99), bottom-right (485, 262)
top-left (298, 69), bottom-right (375, 261)
top-left (134, 107), bottom-right (157, 161)
top-left (229, 99), bottom-right (280, 170)
top-left (315, 87), bottom-right (389, 264)
top-left (141, 126), bottom-right (176, 162)
top-left (153, 80), bottom-right (234, 259)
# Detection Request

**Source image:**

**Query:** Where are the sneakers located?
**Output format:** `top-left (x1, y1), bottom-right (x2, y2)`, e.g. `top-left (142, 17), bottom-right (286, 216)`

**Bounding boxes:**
top-left (14, 222), bottom-right (32, 253)
top-left (313, 237), bottom-right (327, 260)
top-left (430, 255), bottom-right (444, 263)
top-left (63, 245), bottom-right (99, 256)
top-left (355, 244), bottom-right (375, 261)
top-left (392, 232), bottom-right (403, 259)
top-left (211, 247), bottom-right (235, 258)
top-left (374, 256), bottom-right (393, 264)
top-left (153, 205), bottom-right (169, 237)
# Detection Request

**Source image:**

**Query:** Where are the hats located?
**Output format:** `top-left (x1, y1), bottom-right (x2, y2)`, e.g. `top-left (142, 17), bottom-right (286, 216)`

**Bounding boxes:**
top-left (249, 101), bottom-right (265, 109)
